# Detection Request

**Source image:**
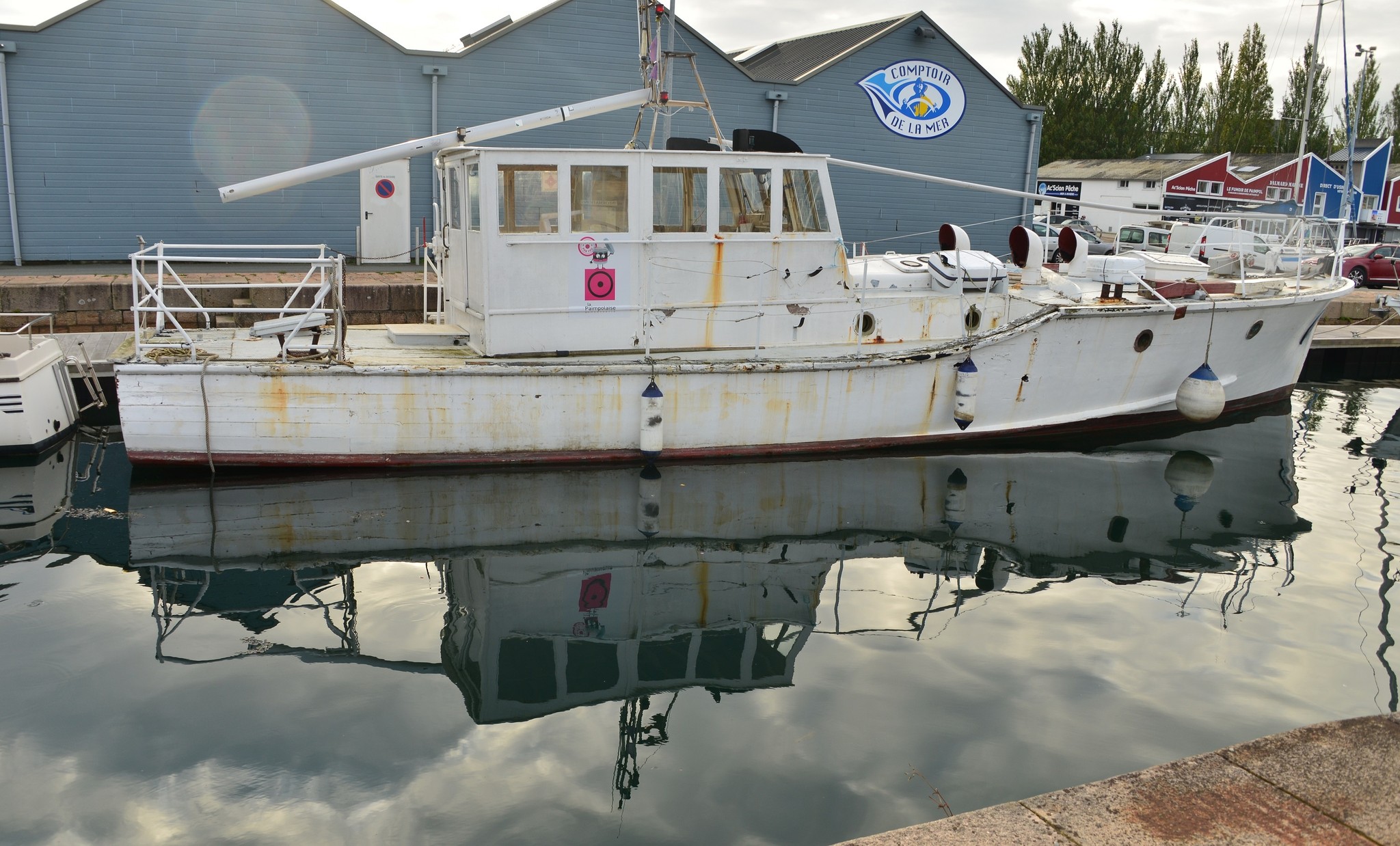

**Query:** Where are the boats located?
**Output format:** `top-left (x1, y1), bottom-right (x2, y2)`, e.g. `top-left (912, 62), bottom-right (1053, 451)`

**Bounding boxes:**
top-left (0, 311), bottom-right (106, 454)
top-left (1268, 243), bottom-right (1336, 273)
top-left (115, 0), bottom-right (1360, 479)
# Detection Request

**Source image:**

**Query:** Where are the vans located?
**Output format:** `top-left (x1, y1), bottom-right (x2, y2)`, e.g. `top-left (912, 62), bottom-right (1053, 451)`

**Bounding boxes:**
top-left (1113, 224), bottom-right (1169, 254)
top-left (1166, 223), bottom-right (1285, 274)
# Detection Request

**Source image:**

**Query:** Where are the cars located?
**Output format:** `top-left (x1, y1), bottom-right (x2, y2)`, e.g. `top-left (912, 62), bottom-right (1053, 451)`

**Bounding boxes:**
top-left (1043, 228), bottom-right (1112, 255)
top-left (1033, 216), bottom-right (1075, 225)
top-left (1060, 219), bottom-right (1096, 236)
top-left (1032, 221), bottom-right (1067, 263)
top-left (1300, 242), bottom-right (1400, 288)
